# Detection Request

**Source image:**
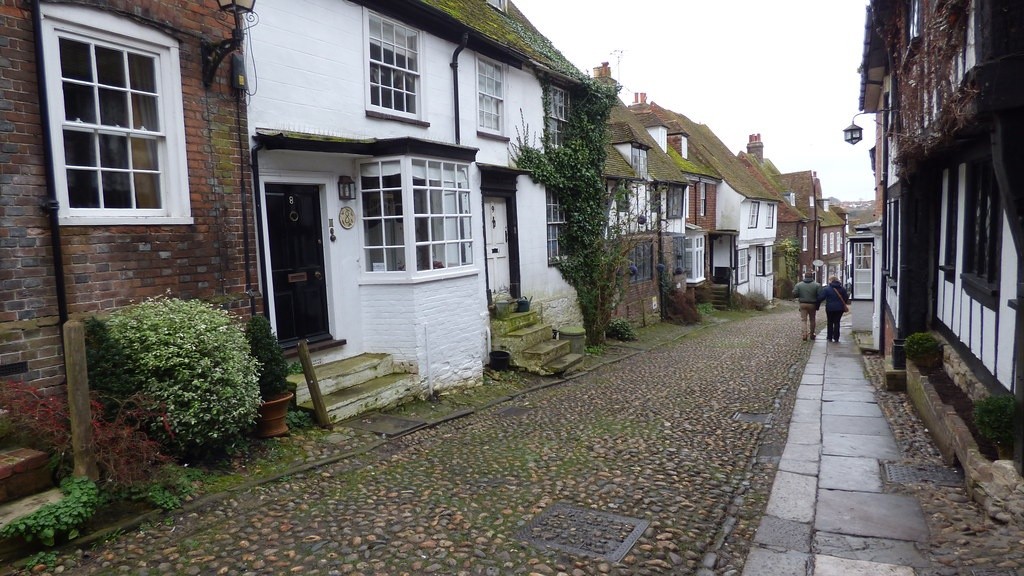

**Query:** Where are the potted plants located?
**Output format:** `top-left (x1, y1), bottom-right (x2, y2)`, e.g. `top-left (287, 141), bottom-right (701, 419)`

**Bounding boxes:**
top-left (971, 391), bottom-right (1022, 453)
top-left (245, 314), bottom-right (296, 437)
top-left (905, 331), bottom-right (943, 371)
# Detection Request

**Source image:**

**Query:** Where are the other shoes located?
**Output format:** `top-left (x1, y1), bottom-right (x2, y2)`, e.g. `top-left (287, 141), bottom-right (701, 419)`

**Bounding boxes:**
top-left (810, 336), bottom-right (815, 340)
top-left (803, 337), bottom-right (807, 341)
top-left (828, 336), bottom-right (832, 341)
top-left (835, 338), bottom-right (838, 342)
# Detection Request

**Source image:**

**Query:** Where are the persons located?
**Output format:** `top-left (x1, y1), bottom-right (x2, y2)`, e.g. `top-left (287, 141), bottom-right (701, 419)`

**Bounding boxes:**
top-left (816, 277), bottom-right (851, 343)
top-left (792, 272), bottom-right (823, 341)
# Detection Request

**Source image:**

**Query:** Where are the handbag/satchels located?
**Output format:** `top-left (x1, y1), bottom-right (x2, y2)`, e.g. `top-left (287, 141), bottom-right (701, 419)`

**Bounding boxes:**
top-left (844, 303), bottom-right (849, 312)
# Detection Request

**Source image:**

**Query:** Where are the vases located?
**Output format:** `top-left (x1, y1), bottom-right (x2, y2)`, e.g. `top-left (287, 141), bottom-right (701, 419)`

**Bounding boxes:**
top-left (489, 351), bottom-right (510, 371)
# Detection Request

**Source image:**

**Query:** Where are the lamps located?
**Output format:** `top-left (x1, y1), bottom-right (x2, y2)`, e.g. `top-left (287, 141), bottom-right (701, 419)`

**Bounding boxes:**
top-left (843, 111), bottom-right (864, 145)
top-left (201, 0), bottom-right (256, 86)
